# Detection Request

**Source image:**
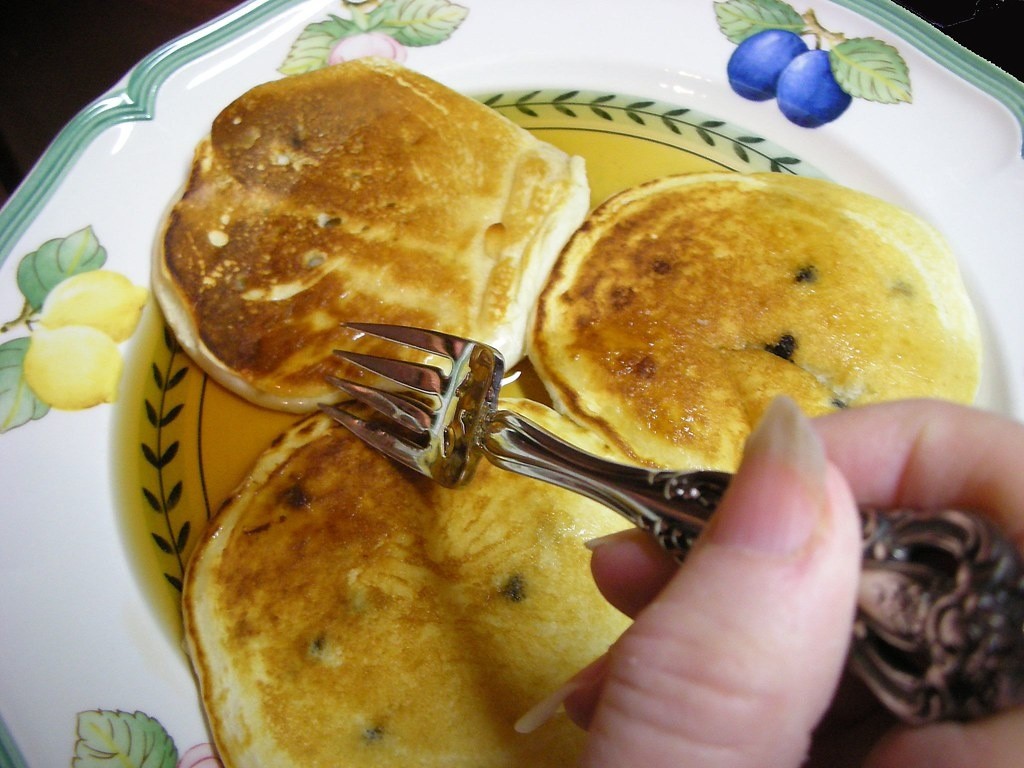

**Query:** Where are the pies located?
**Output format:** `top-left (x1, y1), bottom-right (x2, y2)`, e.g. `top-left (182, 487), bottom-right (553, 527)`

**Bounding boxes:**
top-left (153, 65), bottom-right (996, 768)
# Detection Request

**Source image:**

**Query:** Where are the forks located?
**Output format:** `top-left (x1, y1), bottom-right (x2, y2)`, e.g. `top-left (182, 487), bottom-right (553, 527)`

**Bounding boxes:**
top-left (316, 320), bottom-right (1024, 730)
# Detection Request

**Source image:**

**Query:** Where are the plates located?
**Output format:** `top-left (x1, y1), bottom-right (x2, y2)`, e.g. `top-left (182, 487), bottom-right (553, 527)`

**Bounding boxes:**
top-left (0, 0), bottom-right (1024, 768)
